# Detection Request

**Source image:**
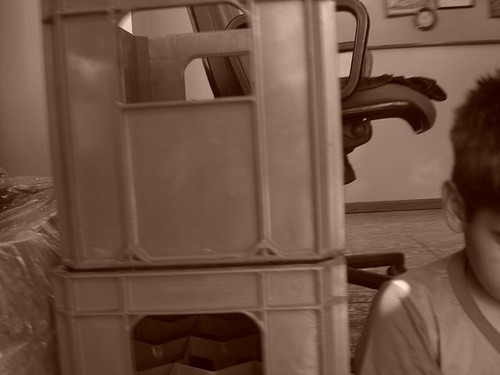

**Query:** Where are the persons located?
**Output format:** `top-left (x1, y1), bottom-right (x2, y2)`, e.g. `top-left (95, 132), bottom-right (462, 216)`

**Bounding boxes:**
top-left (353, 61), bottom-right (500, 375)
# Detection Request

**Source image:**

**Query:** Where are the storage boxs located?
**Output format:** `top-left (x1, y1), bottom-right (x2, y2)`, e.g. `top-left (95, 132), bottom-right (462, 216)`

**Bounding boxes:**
top-left (35, 0), bottom-right (355, 375)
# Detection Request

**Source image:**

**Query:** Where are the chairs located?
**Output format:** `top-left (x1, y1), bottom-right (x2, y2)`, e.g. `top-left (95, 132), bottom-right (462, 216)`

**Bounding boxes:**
top-left (184, 1), bottom-right (448, 298)
